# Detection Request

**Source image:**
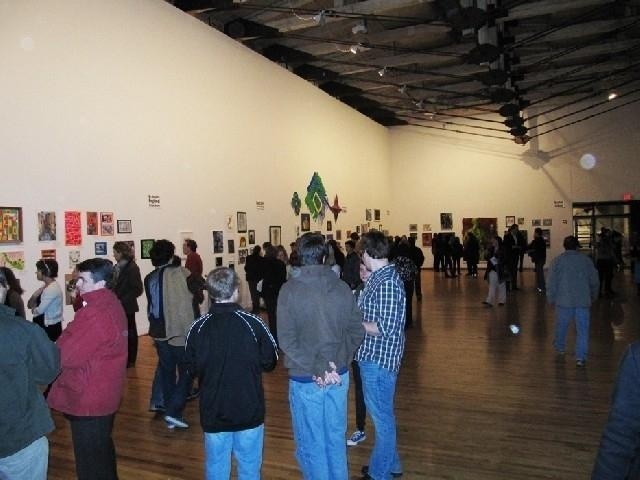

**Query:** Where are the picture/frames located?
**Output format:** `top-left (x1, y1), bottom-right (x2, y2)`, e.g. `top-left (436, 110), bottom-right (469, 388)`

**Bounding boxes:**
top-left (236, 210), bottom-right (247, 234)
top-left (95, 242), bottom-right (108, 255)
top-left (269, 225), bottom-right (283, 247)
top-left (505, 214), bottom-right (515, 228)
top-left (140, 236), bottom-right (154, 261)
top-left (99, 210), bottom-right (115, 236)
top-left (249, 228), bottom-right (256, 245)
top-left (117, 219), bottom-right (132, 234)
top-left (0, 206), bottom-right (24, 243)
top-left (239, 246), bottom-right (247, 264)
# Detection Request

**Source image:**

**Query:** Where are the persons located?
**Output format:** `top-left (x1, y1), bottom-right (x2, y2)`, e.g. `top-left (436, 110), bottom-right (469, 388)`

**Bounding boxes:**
top-left (432, 233), bottom-right (479, 277)
top-left (594, 225), bottom-right (639, 294)
top-left (1, 302), bottom-right (63, 480)
top-left (112, 241), bottom-right (144, 362)
top-left (482, 224), bottom-right (546, 308)
top-left (590, 340), bottom-right (640, 479)
top-left (246, 238), bottom-right (300, 339)
top-left (1, 258), bottom-right (83, 341)
top-left (347, 260), bottom-right (374, 447)
top-left (388, 235), bottom-right (424, 319)
top-left (326, 232), bottom-right (366, 292)
top-left (352, 228), bottom-right (408, 480)
top-left (144, 239), bottom-right (205, 430)
top-left (545, 235), bottom-right (600, 366)
top-left (275, 231), bottom-right (367, 480)
top-left (183, 265), bottom-right (280, 480)
top-left (45, 258), bottom-right (129, 479)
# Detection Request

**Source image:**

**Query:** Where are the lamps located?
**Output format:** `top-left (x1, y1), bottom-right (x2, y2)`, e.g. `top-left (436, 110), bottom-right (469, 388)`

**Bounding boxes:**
top-left (345, 38), bottom-right (376, 57)
top-left (397, 84), bottom-right (409, 93)
top-left (371, 62), bottom-right (390, 82)
top-left (310, 11), bottom-right (372, 36)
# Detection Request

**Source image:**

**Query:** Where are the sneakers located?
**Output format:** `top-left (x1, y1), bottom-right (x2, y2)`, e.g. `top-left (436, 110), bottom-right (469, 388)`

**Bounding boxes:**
top-left (149, 402), bottom-right (165, 412)
top-left (165, 414), bottom-right (188, 429)
top-left (347, 430), bottom-right (366, 445)
top-left (576, 359), bottom-right (584, 367)
top-left (187, 387), bottom-right (199, 399)
